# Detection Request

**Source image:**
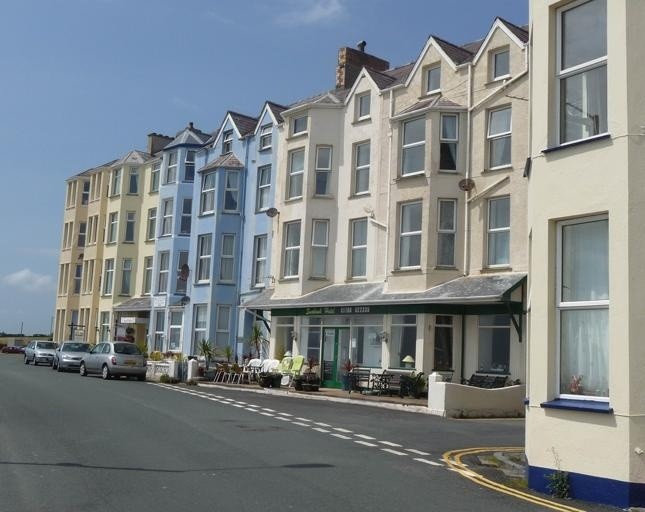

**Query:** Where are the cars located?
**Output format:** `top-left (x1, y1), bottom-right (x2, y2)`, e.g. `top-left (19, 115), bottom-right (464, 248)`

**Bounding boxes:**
top-left (23, 339), bottom-right (59, 365)
top-left (79, 341), bottom-right (148, 381)
top-left (52, 341), bottom-right (92, 372)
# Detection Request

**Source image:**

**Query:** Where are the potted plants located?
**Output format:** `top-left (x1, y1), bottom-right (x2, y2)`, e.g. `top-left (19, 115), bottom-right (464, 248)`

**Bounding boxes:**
top-left (303, 356), bottom-right (317, 383)
top-left (408, 377), bottom-right (426, 399)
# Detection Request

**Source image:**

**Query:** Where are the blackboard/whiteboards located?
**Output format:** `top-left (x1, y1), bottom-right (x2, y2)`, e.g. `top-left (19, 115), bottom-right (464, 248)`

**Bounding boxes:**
top-left (432, 366), bottom-right (455, 383)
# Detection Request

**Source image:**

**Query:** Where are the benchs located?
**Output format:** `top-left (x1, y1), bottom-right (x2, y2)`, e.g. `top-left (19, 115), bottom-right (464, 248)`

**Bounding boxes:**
top-left (371, 370), bottom-right (414, 395)
top-left (462, 373), bottom-right (509, 388)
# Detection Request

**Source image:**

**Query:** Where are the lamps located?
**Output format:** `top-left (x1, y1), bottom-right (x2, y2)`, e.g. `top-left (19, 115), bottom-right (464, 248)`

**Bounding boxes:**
top-left (403, 354), bottom-right (414, 369)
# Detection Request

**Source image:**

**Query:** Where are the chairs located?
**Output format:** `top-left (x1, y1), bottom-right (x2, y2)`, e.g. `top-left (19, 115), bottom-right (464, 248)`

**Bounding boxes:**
top-left (214, 356), bottom-right (304, 389)
